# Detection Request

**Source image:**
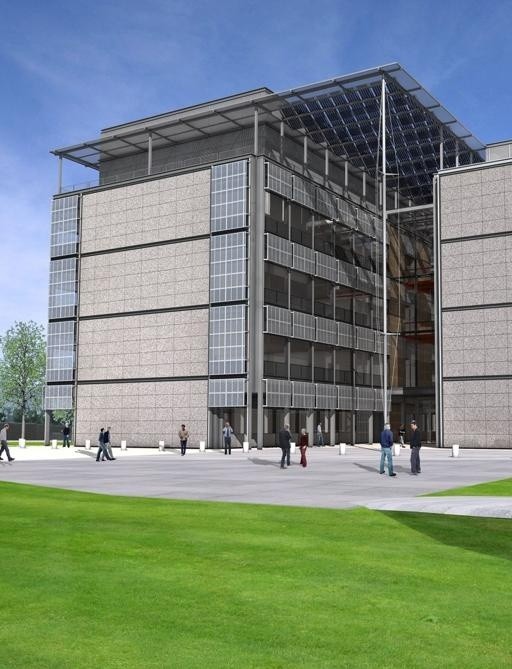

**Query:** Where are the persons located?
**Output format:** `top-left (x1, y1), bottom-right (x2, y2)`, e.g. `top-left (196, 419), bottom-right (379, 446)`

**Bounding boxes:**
top-left (380, 423), bottom-right (396, 476)
top-left (61, 422), bottom-right (70, 448)
top-left (299, 428), bottom-right (308, 467)
top-left (179, 424), bottom-right (189, 456)
top-left (279, 424), bottom-right (291, 469)
top-left (316, 423), bottom-right (324, 447)
top-left (409, 421), bottom-right (421, 476)
top-left (96, 428), bottom-right (112, 461)
top-left (222, 422), bottom-right (233, 454)
top-left (102, 427), bottom-right (116, 461)
top-left (0, 424), bottom-right (14, 462)
top-left (399, 423), bottom-right (406, 447)
